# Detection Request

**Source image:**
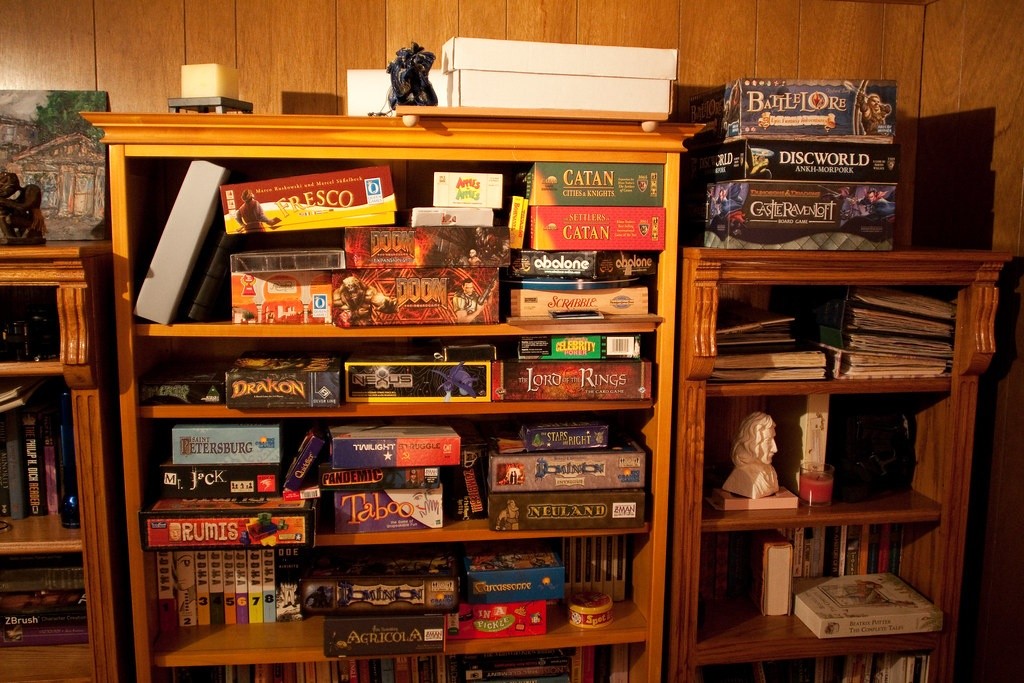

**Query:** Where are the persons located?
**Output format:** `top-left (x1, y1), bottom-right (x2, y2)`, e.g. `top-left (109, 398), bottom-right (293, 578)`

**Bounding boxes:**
top-left (721, 411), bottom-right (780, 499)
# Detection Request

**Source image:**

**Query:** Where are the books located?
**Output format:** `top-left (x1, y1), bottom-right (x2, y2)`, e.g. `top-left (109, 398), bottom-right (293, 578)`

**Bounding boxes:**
top-left (0, 555), bottom-right (89, 645)
top-left (561, 537), bottom-right (628, 602)
top-left (711, 285), bottom-right (955, 380)
top-left (699, 522), bottom-right (944, 639)
top-left (153, 547), bottom-right (302, 632)
top-left (170, 645), bottom-right (629, 683)
top-left (0, 391), bottom-right (80, 529)
top-left (754, 652), bottom-right (929, 683)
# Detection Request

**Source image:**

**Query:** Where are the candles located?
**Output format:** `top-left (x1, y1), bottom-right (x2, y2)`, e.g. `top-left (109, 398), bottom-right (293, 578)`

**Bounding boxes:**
top-left (180, 63), bottom-right (240, 100)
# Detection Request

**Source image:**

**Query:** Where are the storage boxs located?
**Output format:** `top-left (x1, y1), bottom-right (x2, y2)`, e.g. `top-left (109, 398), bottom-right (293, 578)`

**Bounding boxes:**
top-left (149, 40), bottom-right (946, 657)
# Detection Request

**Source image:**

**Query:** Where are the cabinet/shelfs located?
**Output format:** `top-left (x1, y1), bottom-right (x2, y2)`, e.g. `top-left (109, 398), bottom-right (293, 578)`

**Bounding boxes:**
top-left (674, 245), bottom-right (1010, 683)
top-left (1, 242), bottom-right (117, 682)
top-left (84, 110), bottom-right (699, 683)
